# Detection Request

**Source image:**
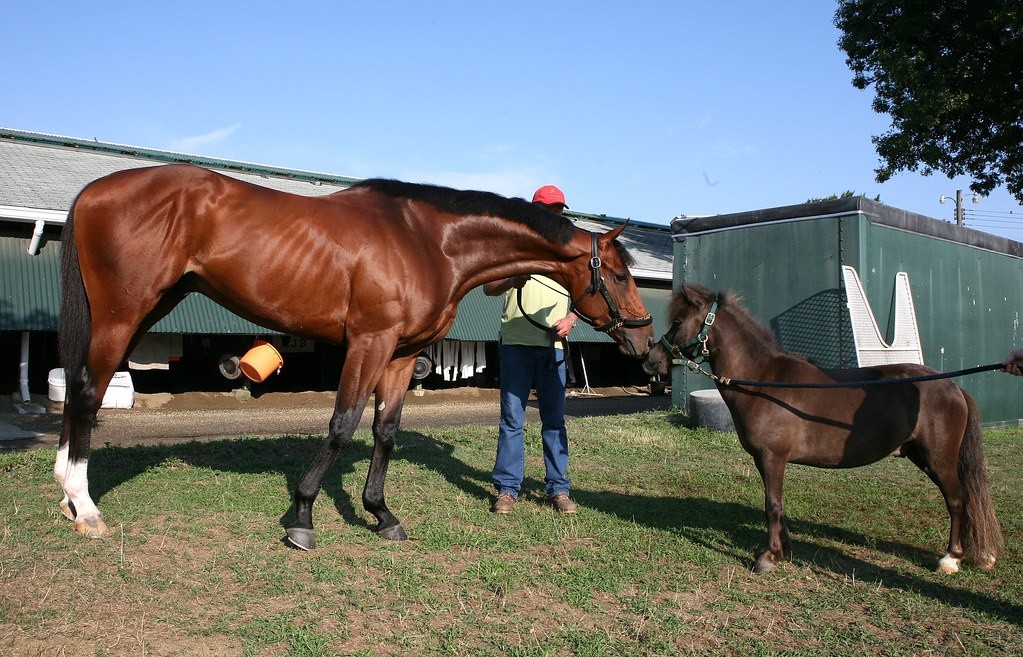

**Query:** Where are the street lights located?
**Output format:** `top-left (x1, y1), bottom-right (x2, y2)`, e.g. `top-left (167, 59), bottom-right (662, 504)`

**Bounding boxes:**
top-left (940, 191), bottom-right (980, 226)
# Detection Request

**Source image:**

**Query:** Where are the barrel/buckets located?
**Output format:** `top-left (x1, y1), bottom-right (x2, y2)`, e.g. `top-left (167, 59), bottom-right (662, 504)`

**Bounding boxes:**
top-left (47, 368), bottom-right (67, 401)
top-left (238, 337), bottom-right (284, 383)
top-left (649, 380), bottom-right (667, 396)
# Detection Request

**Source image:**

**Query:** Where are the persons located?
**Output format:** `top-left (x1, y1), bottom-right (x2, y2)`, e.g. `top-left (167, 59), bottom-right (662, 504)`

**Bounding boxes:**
top-left (483, 185), bottom-right (581, 516)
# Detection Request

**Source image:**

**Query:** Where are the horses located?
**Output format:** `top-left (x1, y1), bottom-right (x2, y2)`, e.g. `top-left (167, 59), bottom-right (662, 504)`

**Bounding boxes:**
top-left (644, 284), bottom-right (1003, 574)
top-left (53, 161), bottom-right (656, 553)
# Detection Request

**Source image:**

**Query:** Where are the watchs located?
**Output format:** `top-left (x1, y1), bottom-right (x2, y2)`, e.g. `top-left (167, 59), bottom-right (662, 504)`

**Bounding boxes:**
top-left (567, 315), bottom-right (576, 328)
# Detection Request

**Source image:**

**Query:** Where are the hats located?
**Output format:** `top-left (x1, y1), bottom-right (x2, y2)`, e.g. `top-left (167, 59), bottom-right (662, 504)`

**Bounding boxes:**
top-left (533, 185), bottom-right (569, 210)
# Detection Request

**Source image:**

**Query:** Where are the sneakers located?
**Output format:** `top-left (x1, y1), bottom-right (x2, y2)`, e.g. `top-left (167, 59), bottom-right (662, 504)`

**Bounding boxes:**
top-left (553, 493), bottom-right (577, 514)
top-left (493, 493), bottom-right (516, 514)
